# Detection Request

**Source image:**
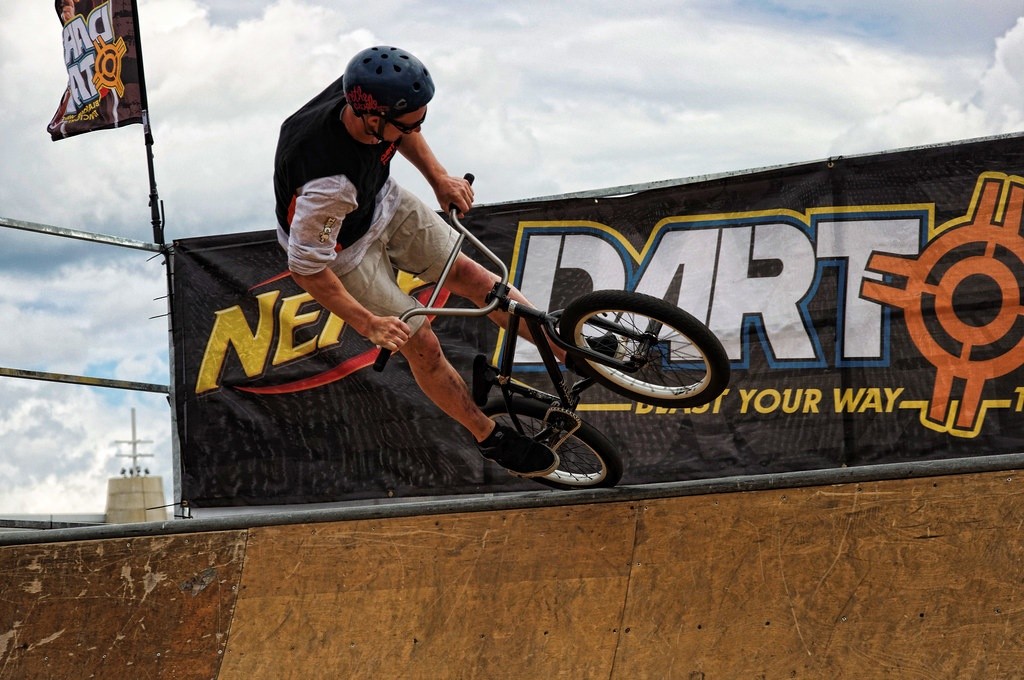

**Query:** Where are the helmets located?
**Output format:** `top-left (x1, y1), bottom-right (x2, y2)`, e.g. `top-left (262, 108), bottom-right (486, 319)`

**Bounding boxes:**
top-left (343, 45), bottom-right (435, 120)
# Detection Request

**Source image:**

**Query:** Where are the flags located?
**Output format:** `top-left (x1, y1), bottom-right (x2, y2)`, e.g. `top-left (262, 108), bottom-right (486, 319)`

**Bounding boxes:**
top-left (46, 0), bottom-right (149, 142)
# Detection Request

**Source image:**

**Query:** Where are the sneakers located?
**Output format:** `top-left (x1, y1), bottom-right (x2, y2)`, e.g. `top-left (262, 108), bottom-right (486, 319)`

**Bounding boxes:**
top-left (472, 427), bottom-right (561, 478)
top-left (585, 333), bottom-right (626, 362)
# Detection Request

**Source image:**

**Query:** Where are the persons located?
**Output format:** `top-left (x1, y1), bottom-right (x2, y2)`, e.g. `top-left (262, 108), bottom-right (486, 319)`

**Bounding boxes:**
top-left (273, 46), bottom-right (619, 478)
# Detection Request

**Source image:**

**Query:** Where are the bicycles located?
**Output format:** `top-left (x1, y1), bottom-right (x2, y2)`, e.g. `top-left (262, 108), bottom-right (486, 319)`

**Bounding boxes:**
top-left (374, 173), bottom-right (734, 491)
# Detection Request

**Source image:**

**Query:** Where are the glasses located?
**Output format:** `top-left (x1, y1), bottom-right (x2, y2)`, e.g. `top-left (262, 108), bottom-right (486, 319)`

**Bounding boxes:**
top-left (387, 109), bottom-right (429, 135)
